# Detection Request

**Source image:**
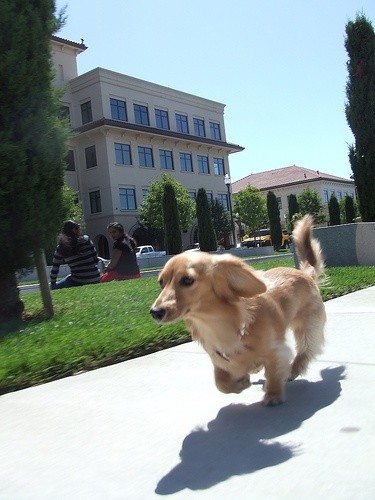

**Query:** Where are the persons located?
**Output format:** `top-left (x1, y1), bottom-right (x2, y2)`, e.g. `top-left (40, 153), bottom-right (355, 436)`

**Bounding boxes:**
top-left (101, 221), bottom-right (141, 282)
top-left (50, 220), bottom-right (101, 290)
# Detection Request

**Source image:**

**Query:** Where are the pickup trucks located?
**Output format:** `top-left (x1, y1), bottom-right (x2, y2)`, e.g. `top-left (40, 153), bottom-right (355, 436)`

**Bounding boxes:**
top-left (135, 245), bottom-right (167, 258)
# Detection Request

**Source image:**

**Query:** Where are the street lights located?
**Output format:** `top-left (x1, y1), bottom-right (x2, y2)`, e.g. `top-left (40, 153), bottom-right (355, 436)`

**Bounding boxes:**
top-left (224, 173), bottom-right (237, 248)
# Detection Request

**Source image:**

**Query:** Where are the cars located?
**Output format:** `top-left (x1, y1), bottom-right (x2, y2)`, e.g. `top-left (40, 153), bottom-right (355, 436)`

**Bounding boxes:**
top-left (241, 228), bottom-right (292, 248)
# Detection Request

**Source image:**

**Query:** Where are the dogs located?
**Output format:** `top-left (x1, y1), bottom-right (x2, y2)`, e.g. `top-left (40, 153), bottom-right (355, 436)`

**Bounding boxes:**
top-left (147, 208), bottom-right (332, 408)
